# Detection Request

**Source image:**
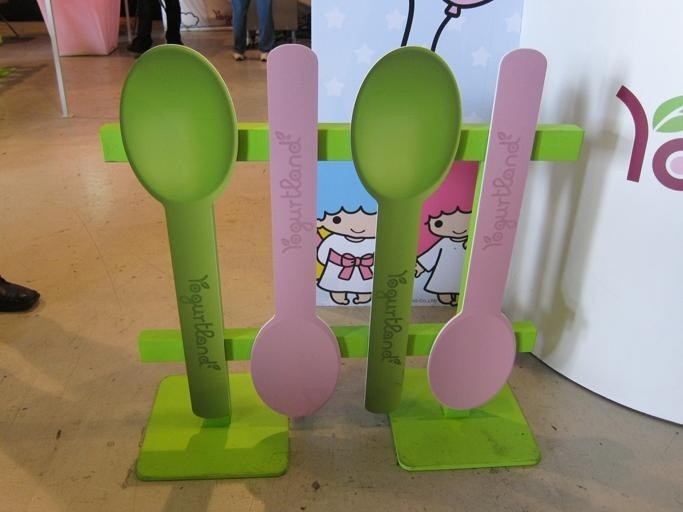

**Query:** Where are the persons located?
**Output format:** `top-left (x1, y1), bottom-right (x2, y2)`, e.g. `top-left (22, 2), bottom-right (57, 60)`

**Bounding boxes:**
top-left (230, 1), bottom-right (278, 63)
top-left (124, 1), bottom-right (184, 54)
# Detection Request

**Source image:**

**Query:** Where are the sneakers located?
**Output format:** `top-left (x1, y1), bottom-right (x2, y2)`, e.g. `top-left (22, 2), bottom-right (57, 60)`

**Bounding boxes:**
top-left (127, 45), bottom-right (147, 53)
top-left (261, 52), bottom-right (268, 61)
top-left (233, 52), bottom-right (244, 60)
